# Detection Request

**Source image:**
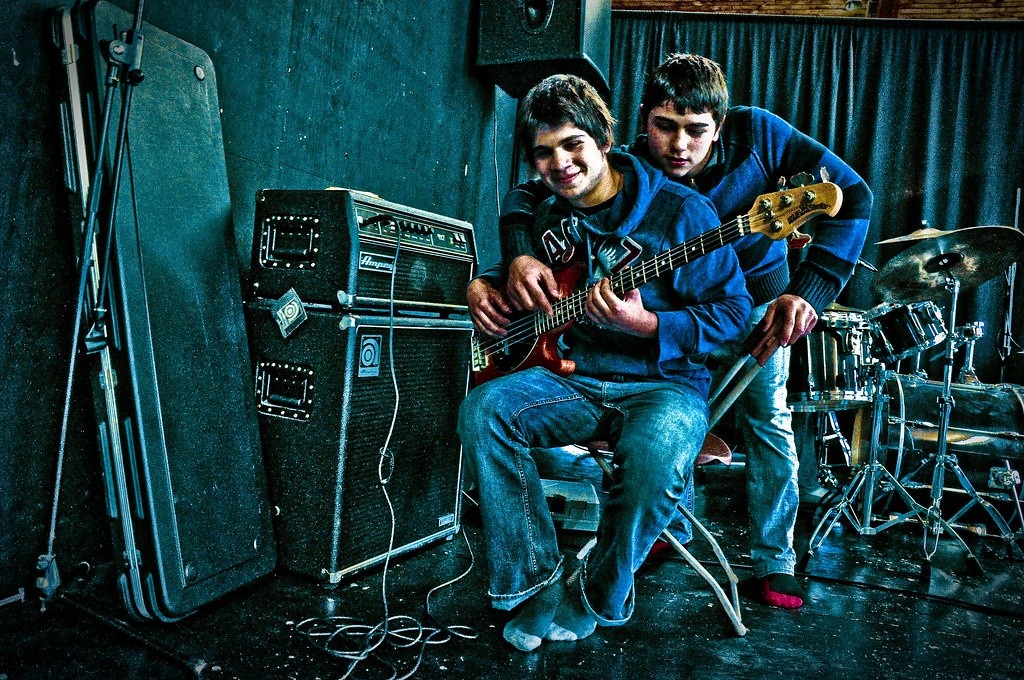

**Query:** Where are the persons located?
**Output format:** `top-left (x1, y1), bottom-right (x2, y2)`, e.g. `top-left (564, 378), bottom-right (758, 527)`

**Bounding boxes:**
top-left (496, 54), bottom-right (874, 606)
top-left (456, 75), bottom-right (751, 650)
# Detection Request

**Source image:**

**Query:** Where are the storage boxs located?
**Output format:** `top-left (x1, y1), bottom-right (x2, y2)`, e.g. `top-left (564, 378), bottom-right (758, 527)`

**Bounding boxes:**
top-left (539, 478), bottom-right (600, 531)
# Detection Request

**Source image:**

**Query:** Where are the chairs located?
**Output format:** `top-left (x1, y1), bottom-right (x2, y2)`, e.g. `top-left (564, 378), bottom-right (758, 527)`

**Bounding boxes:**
top-left (566, 309), bottom-right (785, 637)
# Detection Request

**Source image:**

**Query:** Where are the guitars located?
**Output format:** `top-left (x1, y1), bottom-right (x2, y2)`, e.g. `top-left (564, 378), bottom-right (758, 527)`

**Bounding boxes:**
top-left (470, 165), bottom-right (843, 389)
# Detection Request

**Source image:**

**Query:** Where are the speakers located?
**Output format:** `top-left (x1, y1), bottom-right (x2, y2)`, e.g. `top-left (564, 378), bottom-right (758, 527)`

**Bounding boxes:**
top-left (243, 301), bottom-right (476, 590)
top-left (477, 0), bottom-right (612, 98)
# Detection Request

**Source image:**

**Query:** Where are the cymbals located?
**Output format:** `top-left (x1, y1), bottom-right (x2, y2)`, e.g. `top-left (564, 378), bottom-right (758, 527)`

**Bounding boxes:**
top-left (857, 225), bottom-right (1024, 302)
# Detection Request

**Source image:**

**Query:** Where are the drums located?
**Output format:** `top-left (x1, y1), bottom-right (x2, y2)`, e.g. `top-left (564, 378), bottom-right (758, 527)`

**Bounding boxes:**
top-left (850, 368), bottom-right (1024, 533)
top-left (858, 296), bottom-right (949, 365)
top-left (781, 304), bottom-right (875, 412)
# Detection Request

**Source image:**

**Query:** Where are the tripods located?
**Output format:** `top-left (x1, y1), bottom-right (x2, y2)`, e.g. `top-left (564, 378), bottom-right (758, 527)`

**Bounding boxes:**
top-left (0, 0), bottom-right (226, 680)
top-left (796, 278), bottom-right (1024, 585)
